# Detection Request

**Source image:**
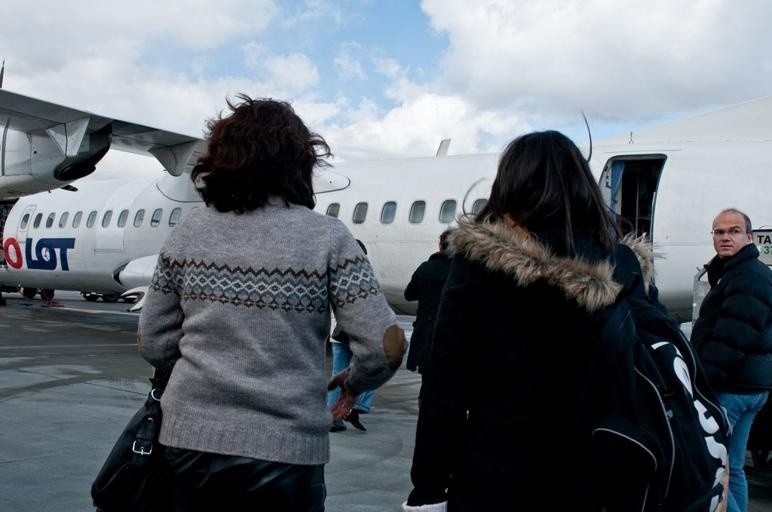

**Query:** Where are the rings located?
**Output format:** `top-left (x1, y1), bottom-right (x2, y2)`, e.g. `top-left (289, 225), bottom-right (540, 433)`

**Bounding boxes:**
top-left (342, 404), bottom-right (346, 408)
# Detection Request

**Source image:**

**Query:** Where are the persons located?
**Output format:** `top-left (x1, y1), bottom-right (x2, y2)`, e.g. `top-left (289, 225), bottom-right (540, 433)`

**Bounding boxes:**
top-left (135, 92), bottom-right (408, 512)
top-left (326, 238), bottom-right (374, 433)
top-left (402, 110), bottom-right (671, 511)
top-left (679, 206), bottom-right (772, 512)
top-left (403, 226), bottom-right (457, 374)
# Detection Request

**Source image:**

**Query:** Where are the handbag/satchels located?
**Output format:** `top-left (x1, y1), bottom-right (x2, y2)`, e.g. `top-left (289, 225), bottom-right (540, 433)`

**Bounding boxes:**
top-left (89, 353), bottom-right (176, 511)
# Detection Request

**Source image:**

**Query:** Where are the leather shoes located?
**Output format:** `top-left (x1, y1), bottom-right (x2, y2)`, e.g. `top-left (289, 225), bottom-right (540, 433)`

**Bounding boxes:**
top-left (328, 415), bottom-right (368, 432)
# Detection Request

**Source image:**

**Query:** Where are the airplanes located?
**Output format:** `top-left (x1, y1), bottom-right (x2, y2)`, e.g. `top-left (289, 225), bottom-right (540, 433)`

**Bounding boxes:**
top-left (1, 88), bottom-right (766, 322)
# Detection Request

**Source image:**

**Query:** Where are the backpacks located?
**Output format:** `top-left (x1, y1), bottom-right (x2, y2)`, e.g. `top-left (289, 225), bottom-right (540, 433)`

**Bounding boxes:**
top-left (520, 254), bottom-right (735, 512)
